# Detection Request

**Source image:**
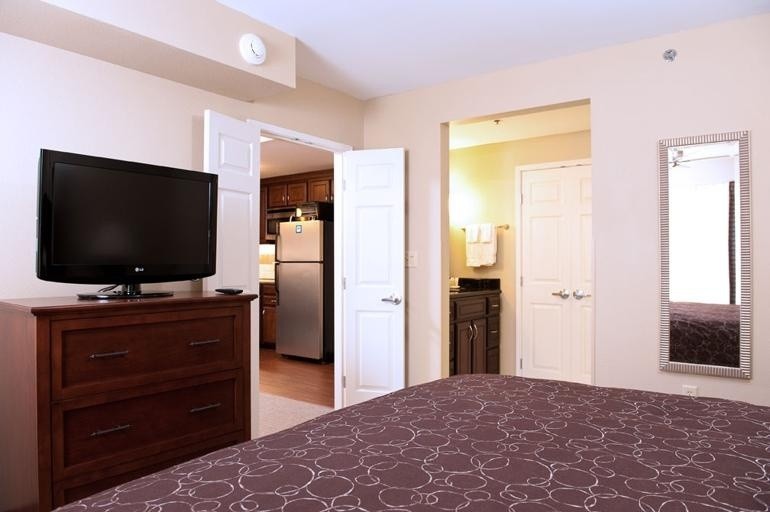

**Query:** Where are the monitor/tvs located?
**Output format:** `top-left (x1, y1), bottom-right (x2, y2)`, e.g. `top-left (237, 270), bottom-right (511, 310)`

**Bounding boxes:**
top-left (36, 148), bottom-right (218, 300)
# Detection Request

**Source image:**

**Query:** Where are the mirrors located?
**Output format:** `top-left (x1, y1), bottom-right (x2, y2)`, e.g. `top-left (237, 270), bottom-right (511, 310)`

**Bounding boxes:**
top-left (657, 130), bottom-right (753, 380)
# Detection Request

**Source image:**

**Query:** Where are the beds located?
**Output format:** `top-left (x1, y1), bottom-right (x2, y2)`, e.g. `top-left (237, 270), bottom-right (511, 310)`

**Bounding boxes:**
top-left (62, 371), bottom-right (769, 511)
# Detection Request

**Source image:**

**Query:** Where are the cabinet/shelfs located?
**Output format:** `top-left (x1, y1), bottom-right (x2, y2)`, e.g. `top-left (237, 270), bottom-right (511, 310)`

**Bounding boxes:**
top-left (449, 290), bottom-right (501, 376)
top-left (258, 277), bottom-right (277, 349)
top-left (308, 177), bottom-right (335, 205)
top-left (268, 180), bottom-right (308, 209)
top-left (265, 209), bottom-right (296, 239)
top-left (0, 290), bottom-right (257, 511)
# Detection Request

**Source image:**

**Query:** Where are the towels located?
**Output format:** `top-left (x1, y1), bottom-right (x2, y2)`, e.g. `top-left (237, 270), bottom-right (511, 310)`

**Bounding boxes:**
top-left (479, 224), bottom-right (492, 243)
top-left (465, 222), bottom-right (480, 244)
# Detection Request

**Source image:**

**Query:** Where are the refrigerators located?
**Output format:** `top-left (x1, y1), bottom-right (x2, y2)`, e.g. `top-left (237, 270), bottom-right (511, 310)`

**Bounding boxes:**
top-left (275, 220), bottom-right (334, 364)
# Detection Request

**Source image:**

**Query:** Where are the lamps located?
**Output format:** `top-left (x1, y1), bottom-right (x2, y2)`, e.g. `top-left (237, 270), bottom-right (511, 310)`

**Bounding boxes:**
top-left (290, 208), bottom-right (303, 223)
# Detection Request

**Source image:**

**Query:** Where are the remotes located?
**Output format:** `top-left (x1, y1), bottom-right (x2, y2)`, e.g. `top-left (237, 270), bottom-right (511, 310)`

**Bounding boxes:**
top-left (215, 288), bottom-right (243, 295)
